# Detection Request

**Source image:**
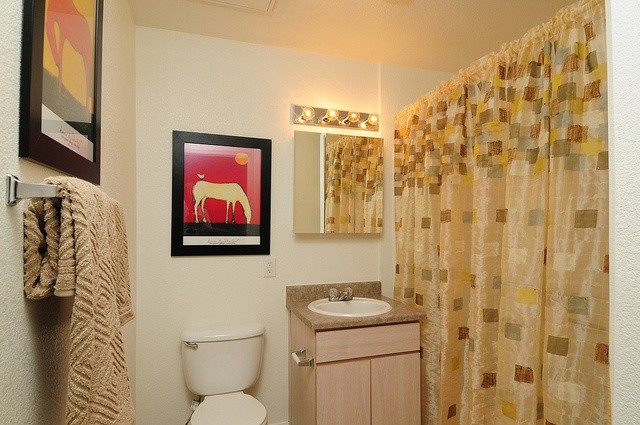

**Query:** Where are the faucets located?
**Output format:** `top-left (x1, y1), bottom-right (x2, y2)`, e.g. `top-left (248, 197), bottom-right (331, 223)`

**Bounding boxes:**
top-left (340, 292), bottom-right (348, 300)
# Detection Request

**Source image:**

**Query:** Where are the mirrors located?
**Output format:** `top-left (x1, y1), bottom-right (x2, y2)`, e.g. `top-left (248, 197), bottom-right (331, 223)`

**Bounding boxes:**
top-left (293, 131), bottom-right (386, 236)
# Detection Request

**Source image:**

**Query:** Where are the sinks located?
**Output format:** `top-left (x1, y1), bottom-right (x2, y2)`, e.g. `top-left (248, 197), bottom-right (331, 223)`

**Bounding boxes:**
top-left (308, 295), bottom-right (392, 317)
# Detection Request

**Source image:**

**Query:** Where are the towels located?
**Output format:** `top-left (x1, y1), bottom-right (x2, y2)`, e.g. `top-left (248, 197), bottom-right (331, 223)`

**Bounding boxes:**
top-left (23, 176), bottom-right (136, 425)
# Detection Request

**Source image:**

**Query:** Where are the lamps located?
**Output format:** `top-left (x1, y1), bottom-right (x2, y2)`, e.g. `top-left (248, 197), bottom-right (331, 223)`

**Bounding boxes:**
top-left (367, 111), bottom-right (381, 128)
top-left (346, 110), bottom-right (361, 123)
top-left (302, 106), bottom-right (318, 123)
top-left (326, 107), bottom-right (340, 122)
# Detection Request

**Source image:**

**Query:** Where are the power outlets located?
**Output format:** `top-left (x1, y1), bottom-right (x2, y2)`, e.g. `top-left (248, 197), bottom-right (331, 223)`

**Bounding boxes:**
top-left (263, 259), bottom-right (276, 279)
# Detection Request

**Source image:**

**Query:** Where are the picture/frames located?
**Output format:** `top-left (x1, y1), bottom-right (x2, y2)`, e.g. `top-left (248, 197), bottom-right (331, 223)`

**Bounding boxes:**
top-left (171, 130), bottom-right (272, 256)
top-left (19, 0), bottom-right (102, 185)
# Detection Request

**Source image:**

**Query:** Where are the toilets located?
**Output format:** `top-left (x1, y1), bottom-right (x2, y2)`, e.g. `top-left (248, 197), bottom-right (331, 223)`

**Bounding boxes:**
top-left (180, 322), bottom-right (268, 425)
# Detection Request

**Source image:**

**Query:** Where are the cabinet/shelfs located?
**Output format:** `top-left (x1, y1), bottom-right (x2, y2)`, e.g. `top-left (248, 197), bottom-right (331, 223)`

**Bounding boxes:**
top-left (288, 309), bottom-right (422, 425)
